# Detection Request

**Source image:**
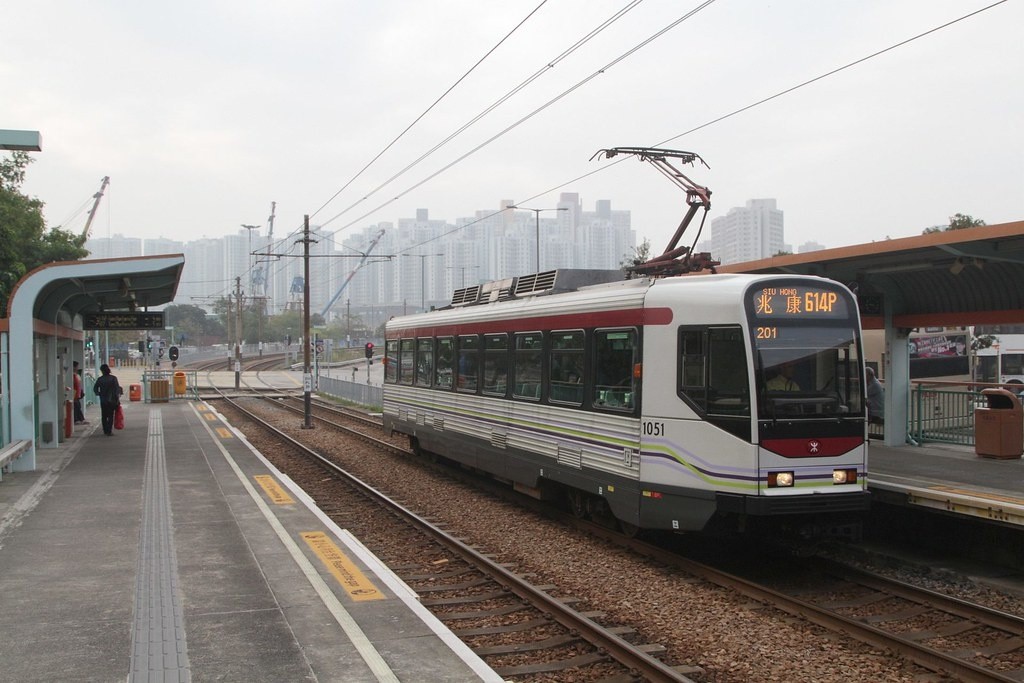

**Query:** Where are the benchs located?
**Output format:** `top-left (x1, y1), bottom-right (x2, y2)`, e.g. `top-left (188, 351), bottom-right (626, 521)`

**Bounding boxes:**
top-left (0, 439), bottom-right (33, 470)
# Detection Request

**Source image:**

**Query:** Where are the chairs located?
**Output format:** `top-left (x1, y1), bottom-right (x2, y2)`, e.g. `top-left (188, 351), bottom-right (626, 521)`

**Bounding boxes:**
top-left (496, 380), bottom-right (582, 404)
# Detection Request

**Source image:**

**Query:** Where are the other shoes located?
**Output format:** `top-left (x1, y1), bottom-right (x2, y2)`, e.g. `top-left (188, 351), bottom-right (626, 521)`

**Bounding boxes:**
top-left (81, 422), bottom-right (89, 425)
top-left (103, 431), bottom-right (114, 436)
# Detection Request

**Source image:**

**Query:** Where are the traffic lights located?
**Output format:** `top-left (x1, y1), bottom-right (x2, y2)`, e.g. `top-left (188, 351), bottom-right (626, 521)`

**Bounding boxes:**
top-left (146, 337), bottom-right (153, 349)
top-left (365, 343), bottom-right (374, 359)
top-left (170, 346), bottom-right (178, 361)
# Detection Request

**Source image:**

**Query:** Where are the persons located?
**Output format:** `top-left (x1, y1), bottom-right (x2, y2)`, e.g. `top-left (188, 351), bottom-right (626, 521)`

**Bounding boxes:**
top-left (866, 367), bottom-right (885, 425)
top-left (73, 361), bottom-right (84, 422)
top-left (93, 364), bottom-right (120, 436)
top-left (953, 336), bottom-right (966, 356)
top-left (763, 353), bottom-right (800, 392)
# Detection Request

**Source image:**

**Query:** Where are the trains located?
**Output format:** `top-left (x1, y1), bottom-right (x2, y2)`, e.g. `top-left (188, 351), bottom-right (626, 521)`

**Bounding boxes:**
top-left (971, 334), bottom-right (1024, 400)
top-left (838, 325), bottom-right (975, 430)
top-left (380, 146), bottom-right (874, 540)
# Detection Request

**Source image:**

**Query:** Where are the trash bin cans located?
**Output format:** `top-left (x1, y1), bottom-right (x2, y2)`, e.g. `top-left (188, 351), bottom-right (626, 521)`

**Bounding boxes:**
top-left (130, 383), bottom-right (141, 401)
top-left (65, 386), bottom-right (73, 438)
top-left (151, 379), bottom-right (169, 403)
top-left (975, 388), bottom-right (1024, 459)
top-left (174, 372), bottom-right (186, 394)
top-left (110, 357), bottom-right (114, 367)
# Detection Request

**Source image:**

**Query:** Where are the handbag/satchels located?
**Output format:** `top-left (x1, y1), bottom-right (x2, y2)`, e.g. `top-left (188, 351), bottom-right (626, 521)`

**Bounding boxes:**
top-left (114, 405), bottom-right (125, 430)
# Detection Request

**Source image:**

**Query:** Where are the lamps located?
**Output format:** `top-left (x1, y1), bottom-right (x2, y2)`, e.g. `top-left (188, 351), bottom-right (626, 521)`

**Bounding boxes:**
top-left (948, 258), bottom-right (965, 275)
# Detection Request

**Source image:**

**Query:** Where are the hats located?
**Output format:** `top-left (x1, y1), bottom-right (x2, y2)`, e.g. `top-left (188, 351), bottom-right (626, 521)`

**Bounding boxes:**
top-left (100, 364), bottom-right (108, 373)
